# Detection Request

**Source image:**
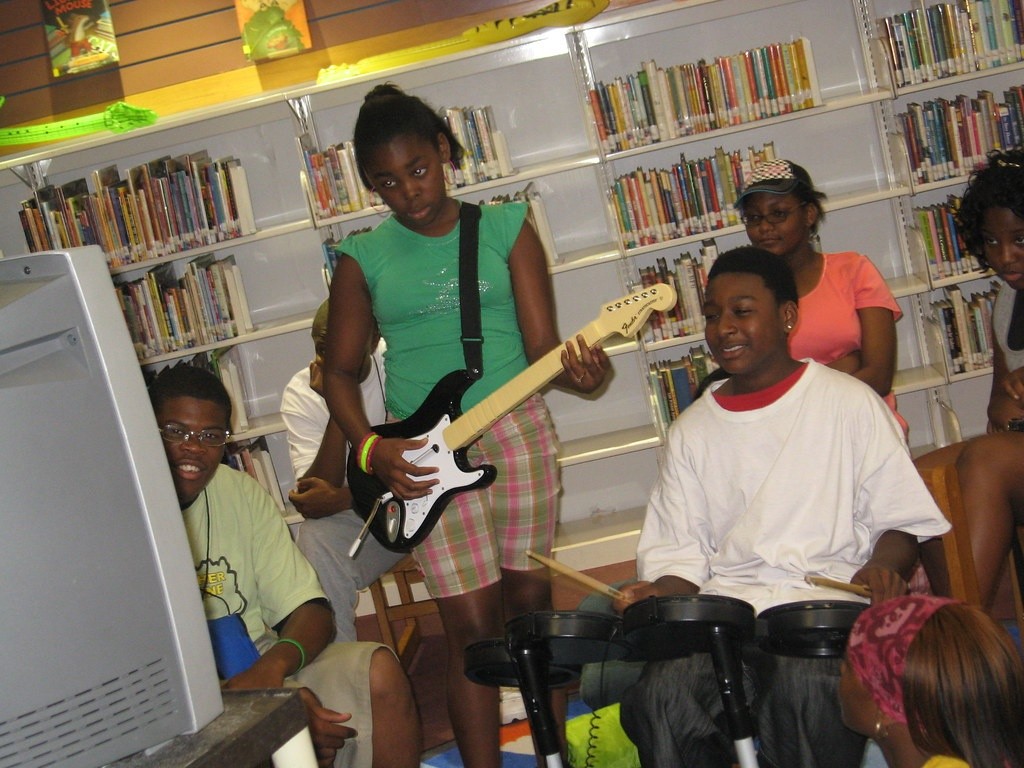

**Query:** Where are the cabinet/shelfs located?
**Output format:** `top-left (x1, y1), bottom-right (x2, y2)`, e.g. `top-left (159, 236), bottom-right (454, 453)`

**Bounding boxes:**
top-left (0, 0), bottom-right (1024, 618)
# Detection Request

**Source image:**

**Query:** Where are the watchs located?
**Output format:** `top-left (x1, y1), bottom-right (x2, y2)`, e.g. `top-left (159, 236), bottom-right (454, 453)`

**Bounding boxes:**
top-left (1007, 418), bottom-right (1024, 432)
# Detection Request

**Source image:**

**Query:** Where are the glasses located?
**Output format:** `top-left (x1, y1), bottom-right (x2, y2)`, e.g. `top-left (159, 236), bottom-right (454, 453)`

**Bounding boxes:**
top-left (159, 424), bottom-right (233, 449)
top-left (739, 198), bottom-right (807, 228)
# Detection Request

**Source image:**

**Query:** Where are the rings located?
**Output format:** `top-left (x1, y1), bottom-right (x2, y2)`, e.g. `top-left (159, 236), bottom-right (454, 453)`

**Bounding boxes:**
top-left (577, 371), bottom-right (586, 382)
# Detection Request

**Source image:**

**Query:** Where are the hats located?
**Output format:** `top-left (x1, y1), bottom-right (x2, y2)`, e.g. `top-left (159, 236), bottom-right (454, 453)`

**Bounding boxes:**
top-left (732, 159), bottom-right (811, 208)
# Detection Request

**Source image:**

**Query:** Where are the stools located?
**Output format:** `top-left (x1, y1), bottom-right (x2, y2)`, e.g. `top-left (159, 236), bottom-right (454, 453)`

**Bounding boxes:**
top-left (370, 554), bottom-right (447, 673)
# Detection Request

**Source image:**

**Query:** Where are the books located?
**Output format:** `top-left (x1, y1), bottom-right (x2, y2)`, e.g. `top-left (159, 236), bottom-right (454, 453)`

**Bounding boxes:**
top-left (912, 194), bottom-right (982, 280)
top-left (637, 238), bottom-right (719, 342)
top-left (147, 345), bottom-right (251, 435)
top-left (442, 105), bottom-right (502, 189)
top-left (612, 140), bottom-right (776, 250)
top-left (873, 0), bottom-right (1024, 87)
top-left (894, 85), bottom-right (1024, 185)
top-left (478, 182), bottom-right (538, 234)
top-left (589, 37), bottom-right (823, 156)
top-left (305, 140), bottom-right (386, 220)
top-left (19, 150), bottom-right (257, 268)
top-left (930, 280), bottom-right (1002, 374)
top-left (649, 344), bottom-right (721, 428)
top-left (114, 253), bottom-right (238, 359)
top-left (323, 226), bottom-right (374, 277)
top-left (222, 436), bottom-right (285, 512)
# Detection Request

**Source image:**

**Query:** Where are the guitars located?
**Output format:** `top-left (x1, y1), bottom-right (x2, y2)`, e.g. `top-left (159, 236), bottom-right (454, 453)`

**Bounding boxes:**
top-left (347, 283), bottom-right (678, 550)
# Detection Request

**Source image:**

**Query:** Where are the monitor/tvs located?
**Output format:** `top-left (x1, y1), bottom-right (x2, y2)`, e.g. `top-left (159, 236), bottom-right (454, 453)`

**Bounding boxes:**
top-left (0, 246), bottom-right (224, 768)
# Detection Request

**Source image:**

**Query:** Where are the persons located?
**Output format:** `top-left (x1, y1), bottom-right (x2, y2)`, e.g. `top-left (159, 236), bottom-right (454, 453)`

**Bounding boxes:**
top-left (836, 593), bottom-right (1024, 768)
top-left (952, 149), bottom-right (1024, 608)
top-left (735, 158), bottom-right (909, 445)
top-left (149, 365), bottom-right (422, 768)
top-left (320, 83), bottom-right (609, 768)
top-left (282, 299), bottom-right (409, 642)
top-left (608, 246), bottom-right (953, 768)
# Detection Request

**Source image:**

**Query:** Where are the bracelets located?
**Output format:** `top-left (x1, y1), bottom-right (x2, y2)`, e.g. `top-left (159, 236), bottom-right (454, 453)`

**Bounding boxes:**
top-left (355, 431), bottom-right (382, 475)
top-left (278, 638), bottom-right (305, 674)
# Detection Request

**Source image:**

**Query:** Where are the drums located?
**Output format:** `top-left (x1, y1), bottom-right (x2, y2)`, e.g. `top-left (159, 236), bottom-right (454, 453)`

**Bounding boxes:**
top-left (503, 610), bottom-right (632, 667)
top-left (462, 638), bottom-right (581, 688)
top-left (624, 594), bottom-right (755, 654)
top-left (755, 600), bottom-right (871, 659)
